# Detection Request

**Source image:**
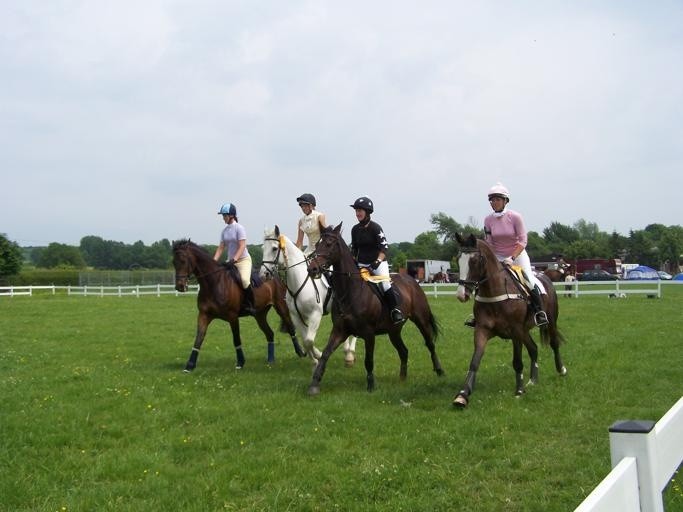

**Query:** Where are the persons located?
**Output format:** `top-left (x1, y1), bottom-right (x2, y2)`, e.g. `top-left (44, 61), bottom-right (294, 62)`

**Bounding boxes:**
top-left (563, 270), bottom-right (576, 297)
top-left (465, 183), bottom-right (548, 328)
top-left (211, 202), bottom-right (257, 314)
top-left (347, 197), bottom-right (404, 324)
top-left (292, 192), bottom-right (328, 258)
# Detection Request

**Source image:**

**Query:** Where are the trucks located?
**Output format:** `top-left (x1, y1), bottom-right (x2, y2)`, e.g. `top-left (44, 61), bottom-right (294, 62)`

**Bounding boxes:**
top-left (405, 259), bottom-right (451, 283)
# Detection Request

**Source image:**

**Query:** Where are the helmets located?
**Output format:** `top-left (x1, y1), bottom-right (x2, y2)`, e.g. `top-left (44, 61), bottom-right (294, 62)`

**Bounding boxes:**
top-left (297, 194), bottom-right (316, 206)
top-left (349, 196), bottom-right (373, 212)
top-left (218, 204), bottom-right (238, 214)
top-left (487, 185), bottom-right (510, 203)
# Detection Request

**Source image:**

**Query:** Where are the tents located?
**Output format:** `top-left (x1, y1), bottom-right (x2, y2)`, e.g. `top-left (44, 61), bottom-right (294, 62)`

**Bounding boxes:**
top-left (625, 265), bottom-right (661, 281)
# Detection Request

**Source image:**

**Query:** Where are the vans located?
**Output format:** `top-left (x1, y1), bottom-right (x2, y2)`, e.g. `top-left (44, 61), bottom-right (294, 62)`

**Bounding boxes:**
top-left (581, 269), bottom-right (621, 281)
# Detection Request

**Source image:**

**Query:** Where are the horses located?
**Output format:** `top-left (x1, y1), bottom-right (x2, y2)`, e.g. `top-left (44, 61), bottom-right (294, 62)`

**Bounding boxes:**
top-left (170, 237), bottom-right (307, 373)
top-left (258, 225), bottom-right (358, 369)
top-left (305, 220), bottom-right (446, 395)
top-left (452, 231), bottom-right (568, 408)
top-left (431, 272), bottom-right (446, 284)
top-left (544, 264), bottom-right (572, 282)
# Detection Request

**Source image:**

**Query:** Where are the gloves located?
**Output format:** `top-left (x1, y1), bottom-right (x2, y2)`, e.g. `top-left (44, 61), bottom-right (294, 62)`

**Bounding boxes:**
top-left (503, 256), bottom-right (513, 266)
top-left (372, 259), bottom-right (381, 268)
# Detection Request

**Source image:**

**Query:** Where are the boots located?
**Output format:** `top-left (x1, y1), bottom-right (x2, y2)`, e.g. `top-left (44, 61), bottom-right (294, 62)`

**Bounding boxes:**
top-left (530, 285), bottom-right (546, 324)
top-left (384, 286), bottom-right (403, 322)
top-left (465, 317), bottom-right (476, 326)
top-left (244, 285), bottom-right (256, 317)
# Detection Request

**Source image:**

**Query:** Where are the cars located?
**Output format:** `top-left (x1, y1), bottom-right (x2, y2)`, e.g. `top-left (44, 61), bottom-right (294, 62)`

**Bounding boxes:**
top-left (657, 270), bottom-right (673, 280)
